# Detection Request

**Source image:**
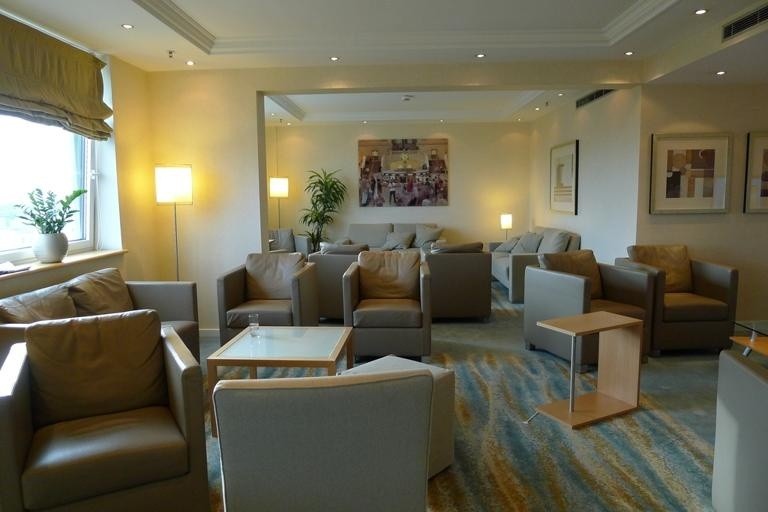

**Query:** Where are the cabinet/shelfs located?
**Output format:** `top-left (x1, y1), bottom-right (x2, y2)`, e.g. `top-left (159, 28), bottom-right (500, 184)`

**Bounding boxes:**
top-left (710, 318), bottom-right (768, 511)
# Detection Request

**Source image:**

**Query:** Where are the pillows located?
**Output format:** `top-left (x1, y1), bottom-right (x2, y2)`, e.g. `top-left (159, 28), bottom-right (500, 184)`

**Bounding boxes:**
top-left (511, 232), bottom-right (545, 256)
top-left (494, 235), bottom-right (520, 253)
top-left (411, 224), bottom-right (445, 249)
top-left (537, 231), bottom-right (572, 254)
top-left (381, 232), bottom-right (416, 249)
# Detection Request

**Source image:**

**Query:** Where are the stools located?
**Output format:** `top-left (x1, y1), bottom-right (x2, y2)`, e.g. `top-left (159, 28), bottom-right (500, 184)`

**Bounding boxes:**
top-left (341, 351), bottom-right (456, 485)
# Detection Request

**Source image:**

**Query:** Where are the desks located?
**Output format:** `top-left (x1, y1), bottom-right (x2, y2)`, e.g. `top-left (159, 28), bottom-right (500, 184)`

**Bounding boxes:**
top-left (533, 310), bottom-right (644, 430)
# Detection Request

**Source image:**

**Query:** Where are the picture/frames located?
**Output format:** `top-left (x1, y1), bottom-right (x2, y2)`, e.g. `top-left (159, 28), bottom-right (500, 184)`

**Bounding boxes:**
top-left (741, 129), bottom-right (768, 215)
top-left (548, 138), bottom-right (581, 216)
top-left (648, 129), bottom-right (734, 216)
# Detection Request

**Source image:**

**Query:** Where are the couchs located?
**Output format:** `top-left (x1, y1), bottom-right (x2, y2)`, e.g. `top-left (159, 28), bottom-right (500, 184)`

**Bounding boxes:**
top-left (307, 240), bottom-right (494, 324)
top-left (1, 267), bottom-right (201, 376)
top-left (333, 222), bottom-right (448, 247)
top-left (486, 223), bottom-right (581, 306)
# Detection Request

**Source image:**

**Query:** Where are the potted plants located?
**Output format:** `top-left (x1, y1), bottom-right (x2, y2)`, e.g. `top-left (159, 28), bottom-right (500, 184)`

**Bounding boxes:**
top-left (10, 182), bottom-right (88, 268)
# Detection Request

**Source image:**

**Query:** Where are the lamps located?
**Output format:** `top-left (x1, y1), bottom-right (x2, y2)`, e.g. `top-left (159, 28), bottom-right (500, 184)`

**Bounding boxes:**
top-left (266, 177), bottom-right (291, 229)
top-left (151, 163), bottom-right (193, 283)
top-left (500, 213), bottom-right (513, 240)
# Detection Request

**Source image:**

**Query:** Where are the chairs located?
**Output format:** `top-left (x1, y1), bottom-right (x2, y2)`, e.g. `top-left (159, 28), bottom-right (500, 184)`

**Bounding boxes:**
top-left (208, 368), bottom-right (434, 512)
top-left (341, 247), bottom-right (434, 364)
top-left (615, 243), bottom-right (740, 358)
top-left (521, 249), bottom-right (656, 373)
top-left (1, 308), bottom-right (211, 511)
top-left (267, 227), bottom-right (313, 260)
top-left (215, 250), bottom-right (321, 349)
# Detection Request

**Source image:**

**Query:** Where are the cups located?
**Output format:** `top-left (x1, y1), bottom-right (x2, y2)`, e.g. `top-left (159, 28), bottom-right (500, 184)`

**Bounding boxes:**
top-left (431, 243), bottom-right (440, 255)
top-left (248, 314), bottom-right (259, 336)
